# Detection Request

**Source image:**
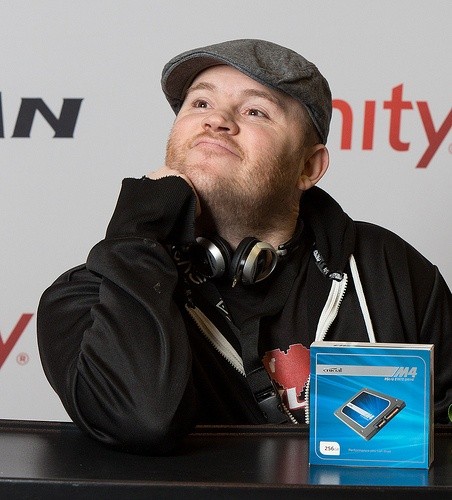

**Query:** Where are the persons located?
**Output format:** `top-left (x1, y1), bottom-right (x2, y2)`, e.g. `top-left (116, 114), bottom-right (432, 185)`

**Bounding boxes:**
top-left (36, 38), bottom-right (452, 449)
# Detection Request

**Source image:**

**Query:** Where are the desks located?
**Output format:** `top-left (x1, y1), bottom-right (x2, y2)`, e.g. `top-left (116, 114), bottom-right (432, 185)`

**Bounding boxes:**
top-left (0, 419), bottom-right (452, 500)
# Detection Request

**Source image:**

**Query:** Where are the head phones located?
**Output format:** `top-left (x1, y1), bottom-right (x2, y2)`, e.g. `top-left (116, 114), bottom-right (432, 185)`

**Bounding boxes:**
top-left (188, 229), bottom-right (304, 290)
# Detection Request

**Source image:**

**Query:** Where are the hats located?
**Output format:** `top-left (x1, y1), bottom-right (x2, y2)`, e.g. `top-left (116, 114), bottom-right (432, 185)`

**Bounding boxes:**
top-left (159, 34), bottom-right (331, 148)
